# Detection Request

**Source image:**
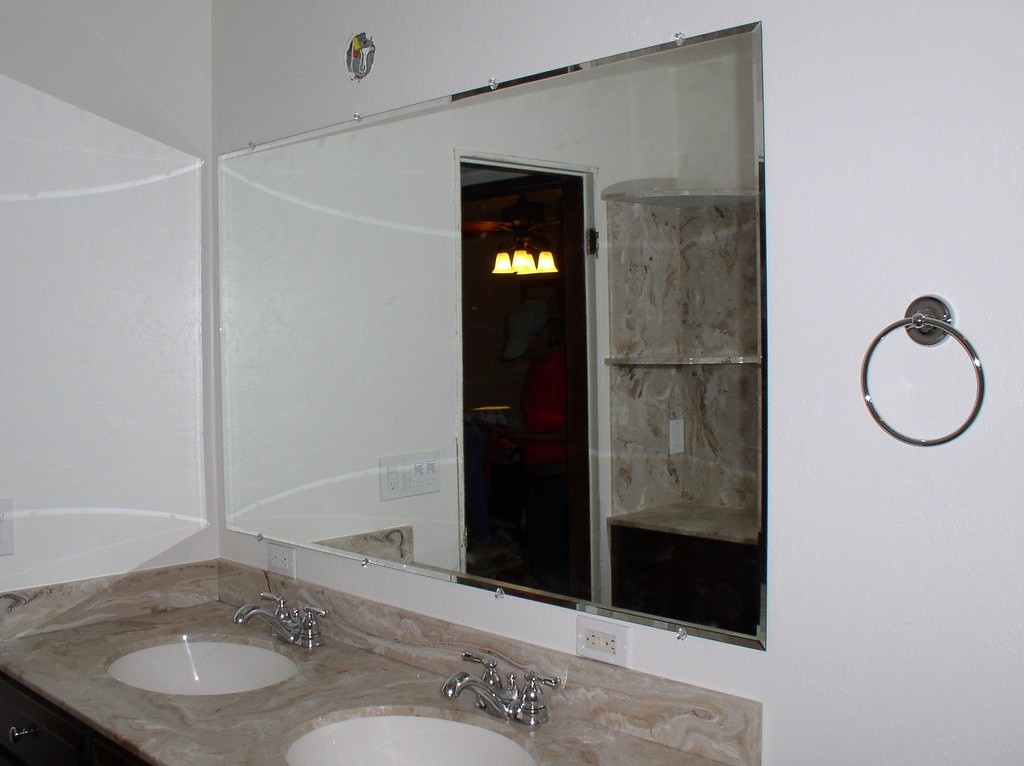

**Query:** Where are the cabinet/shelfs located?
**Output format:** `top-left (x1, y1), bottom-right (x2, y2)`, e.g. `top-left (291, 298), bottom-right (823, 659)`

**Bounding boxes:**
top-left (0, 670), bottom-right (152, 766)
top-left (608, 189), bottom-right (764, 547)
top-left (612, 524), bottom-right (762, 636)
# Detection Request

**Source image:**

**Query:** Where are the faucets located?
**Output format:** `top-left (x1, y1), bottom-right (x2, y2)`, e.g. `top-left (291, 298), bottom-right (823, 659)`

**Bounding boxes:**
top-left (441, 651), bottom-right (561, 726)
top-left (232, 591), bottom-right (330, 647)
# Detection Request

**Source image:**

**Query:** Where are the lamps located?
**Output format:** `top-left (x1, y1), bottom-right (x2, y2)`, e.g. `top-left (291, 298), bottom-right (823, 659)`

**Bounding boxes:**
top-left (489, 198), bottom-right (562, 275)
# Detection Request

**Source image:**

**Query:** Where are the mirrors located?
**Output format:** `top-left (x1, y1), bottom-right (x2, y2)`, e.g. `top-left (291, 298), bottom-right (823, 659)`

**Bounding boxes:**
top-left (221, 21), bottom-right (767, 653)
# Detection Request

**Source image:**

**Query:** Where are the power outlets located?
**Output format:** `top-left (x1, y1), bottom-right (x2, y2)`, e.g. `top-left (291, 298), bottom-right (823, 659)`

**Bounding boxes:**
top-left (379, 448), bottom-right (441, 501)
top-left (575, 615), bottom-right (632, 667)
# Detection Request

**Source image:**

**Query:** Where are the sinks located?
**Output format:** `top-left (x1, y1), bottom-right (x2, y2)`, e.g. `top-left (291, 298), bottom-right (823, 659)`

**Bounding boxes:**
top-left (282, 703), bottom-right (541, 766)
top-left (104, 631), bottom-right (300, 696)
